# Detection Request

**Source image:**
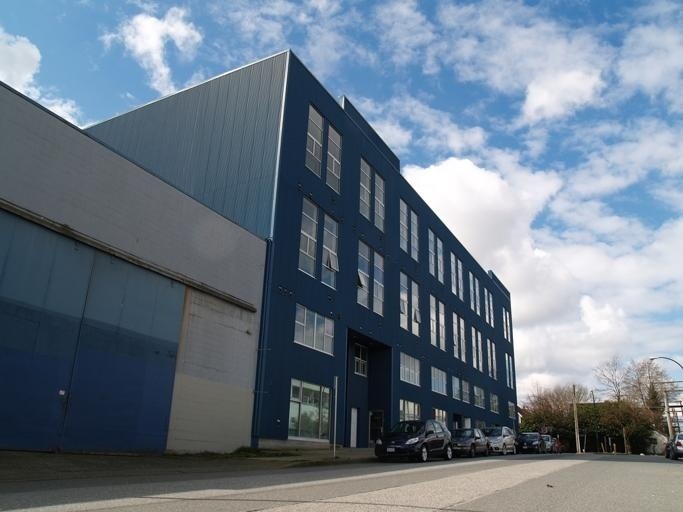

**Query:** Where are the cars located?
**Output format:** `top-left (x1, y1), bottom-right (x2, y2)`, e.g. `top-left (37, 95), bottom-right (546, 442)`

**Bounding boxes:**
top-left (375, 419), bottom-right (562, 463)
top-left (664, 432), bottom-right (683, 460)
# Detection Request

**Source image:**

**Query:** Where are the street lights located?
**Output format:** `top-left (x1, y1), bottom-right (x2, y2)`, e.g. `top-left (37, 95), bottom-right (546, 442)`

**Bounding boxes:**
top-left (649, 356), bottom-right (683, 369)
